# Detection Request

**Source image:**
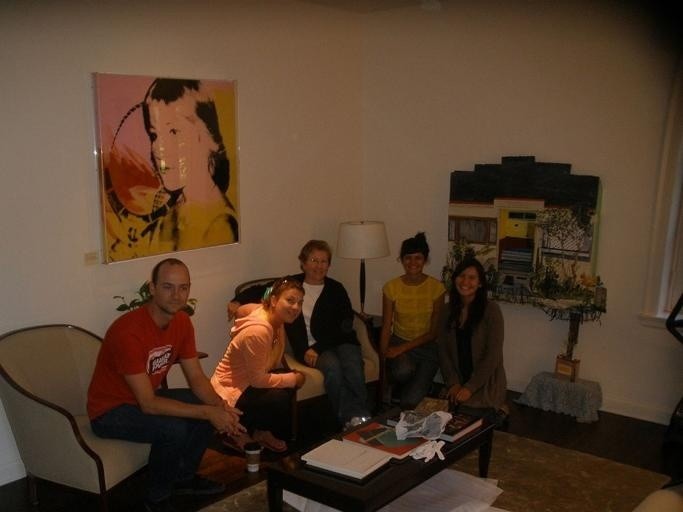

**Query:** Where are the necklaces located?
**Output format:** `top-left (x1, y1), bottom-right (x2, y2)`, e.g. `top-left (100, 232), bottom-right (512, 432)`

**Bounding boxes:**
top-left (268, 322), bottom-right (280, 346)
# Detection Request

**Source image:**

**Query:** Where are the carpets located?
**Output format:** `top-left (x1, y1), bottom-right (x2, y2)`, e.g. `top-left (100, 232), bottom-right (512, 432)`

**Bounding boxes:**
top-left (198, 431), bottom-right (673, 512)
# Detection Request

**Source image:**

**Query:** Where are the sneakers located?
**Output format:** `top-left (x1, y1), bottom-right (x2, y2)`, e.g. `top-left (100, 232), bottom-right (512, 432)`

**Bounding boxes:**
top-left (216, 427), bottom-right (287, 455)
top-left (172, 475), bottom-right (225, 497)
top-left (142, 486), bottom-right (181, 512)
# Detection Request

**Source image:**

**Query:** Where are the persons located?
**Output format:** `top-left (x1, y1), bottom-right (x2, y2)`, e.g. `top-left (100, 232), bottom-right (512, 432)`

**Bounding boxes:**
top-left (436, 257), bottom-right (510, 432)
top-left (284, 240), bottom-right (372, 432)
top-left (378, 232), bottom-right (447, 411)
top-left (86, 258), bottom-right (248, 511)
top-left (210, 277), bottom-right (305, 454)
top-left (109, 77), bottom-right (238, 261)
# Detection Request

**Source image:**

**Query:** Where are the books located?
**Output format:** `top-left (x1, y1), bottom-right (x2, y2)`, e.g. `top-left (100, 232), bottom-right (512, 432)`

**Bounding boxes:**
top-left (342, 421), bottom-right (431, 461)
top-left (385, 408), bottom-right (484, 443)
top-left (301, 438), bottom-right (391, 480)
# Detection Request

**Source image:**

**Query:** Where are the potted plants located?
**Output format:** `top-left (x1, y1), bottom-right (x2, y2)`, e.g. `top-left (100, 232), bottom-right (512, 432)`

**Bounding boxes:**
top-left (528, 208), bottom-right (606, 379)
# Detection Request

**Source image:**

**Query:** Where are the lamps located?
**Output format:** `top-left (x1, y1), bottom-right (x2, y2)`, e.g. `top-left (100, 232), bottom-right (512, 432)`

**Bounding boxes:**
top-left (336, 219), bottom-right (390, 321)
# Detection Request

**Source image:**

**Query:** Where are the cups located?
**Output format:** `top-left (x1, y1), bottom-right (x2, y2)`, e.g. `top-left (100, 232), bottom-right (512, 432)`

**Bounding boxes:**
top-left (243, 441), bottom-right (262, 474)
top-left (594, 286), bottom-right (606, 308)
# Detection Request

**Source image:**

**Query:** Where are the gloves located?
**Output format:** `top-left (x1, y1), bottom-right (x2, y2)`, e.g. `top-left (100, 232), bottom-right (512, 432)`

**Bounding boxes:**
top-left (409, 440), bottom-right (446, 463)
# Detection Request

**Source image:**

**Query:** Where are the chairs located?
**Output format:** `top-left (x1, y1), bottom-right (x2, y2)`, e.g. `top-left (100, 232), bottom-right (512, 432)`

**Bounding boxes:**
top-left (0, 324), bottom-right (154, 512)
top-left (236, 276), bottom-right (383, 441)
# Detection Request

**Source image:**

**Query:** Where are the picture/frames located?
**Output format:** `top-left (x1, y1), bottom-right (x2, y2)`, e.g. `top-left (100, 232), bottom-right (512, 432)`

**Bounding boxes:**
top-left (93, 72), bottom-right (242, 266)
